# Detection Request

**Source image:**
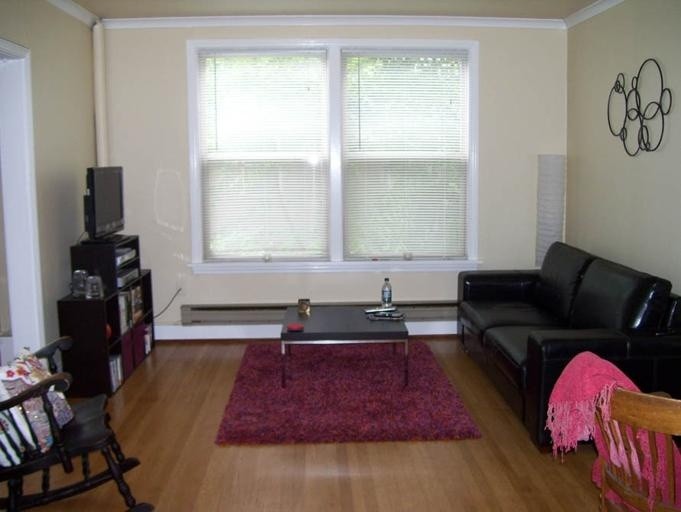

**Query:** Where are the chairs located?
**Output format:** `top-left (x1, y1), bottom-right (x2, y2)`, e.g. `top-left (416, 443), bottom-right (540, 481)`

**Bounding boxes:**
top-left (0, 336), bottom-right (155, 511)
top-left (590, 386), bottom-right (681, 511)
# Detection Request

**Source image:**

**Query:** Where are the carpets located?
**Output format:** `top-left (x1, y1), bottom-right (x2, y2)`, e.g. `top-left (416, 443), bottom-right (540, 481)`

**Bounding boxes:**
top-left (216, 336), bottom-right (482, 446)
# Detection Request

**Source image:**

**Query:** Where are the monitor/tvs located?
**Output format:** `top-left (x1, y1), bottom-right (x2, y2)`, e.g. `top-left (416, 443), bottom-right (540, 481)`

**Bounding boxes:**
top-left (82, 166), bottom-right (130, 245)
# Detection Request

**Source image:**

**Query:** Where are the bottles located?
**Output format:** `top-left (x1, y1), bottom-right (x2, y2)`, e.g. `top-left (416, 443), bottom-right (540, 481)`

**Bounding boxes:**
top-left (298, 299), bottom-right (310, 315)
top-left (381, 278), bottom-right (392, 306)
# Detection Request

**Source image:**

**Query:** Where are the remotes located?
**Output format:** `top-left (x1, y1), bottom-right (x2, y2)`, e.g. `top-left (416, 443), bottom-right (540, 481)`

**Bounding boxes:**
top-left (368, 312), bottom-right (406, 321)
top-left (364, 306), bottom-right (396, 313)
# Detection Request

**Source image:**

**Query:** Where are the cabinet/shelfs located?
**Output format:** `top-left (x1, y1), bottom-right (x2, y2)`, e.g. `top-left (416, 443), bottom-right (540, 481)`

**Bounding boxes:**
top-left (56, 236), bottom-right (156, 398)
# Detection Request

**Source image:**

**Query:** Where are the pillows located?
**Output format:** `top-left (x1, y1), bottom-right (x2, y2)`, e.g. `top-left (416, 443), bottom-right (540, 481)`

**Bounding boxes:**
top-left (0, 350), bottom-right (74, 466)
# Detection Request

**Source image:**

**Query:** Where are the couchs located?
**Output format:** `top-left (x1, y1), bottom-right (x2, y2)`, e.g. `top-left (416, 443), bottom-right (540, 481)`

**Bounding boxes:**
top-left (458, 241), bottom-right (680, 453)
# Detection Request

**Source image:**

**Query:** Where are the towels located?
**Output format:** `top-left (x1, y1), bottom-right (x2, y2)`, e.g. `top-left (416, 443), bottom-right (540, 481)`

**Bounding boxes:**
top-left (545, 347), bottom-right (681, 512)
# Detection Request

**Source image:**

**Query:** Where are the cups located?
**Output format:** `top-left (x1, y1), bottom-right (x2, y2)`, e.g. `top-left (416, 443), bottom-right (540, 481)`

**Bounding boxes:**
top-left (72, 269), bottom-right (104, 302)
